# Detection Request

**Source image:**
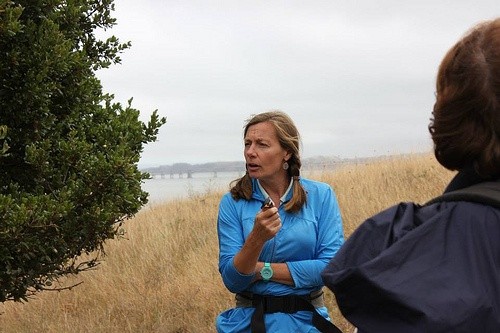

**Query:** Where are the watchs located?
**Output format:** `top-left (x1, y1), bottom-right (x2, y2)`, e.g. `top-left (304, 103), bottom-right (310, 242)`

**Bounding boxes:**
top-left (260, 262), bottom-right (273, 281)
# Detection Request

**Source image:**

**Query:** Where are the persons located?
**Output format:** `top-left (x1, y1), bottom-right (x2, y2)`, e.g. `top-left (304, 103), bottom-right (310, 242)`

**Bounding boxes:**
top-left (215, 111), bottom-right (344, 333)
top-left (321, 17), bottom-right (500, 333)
